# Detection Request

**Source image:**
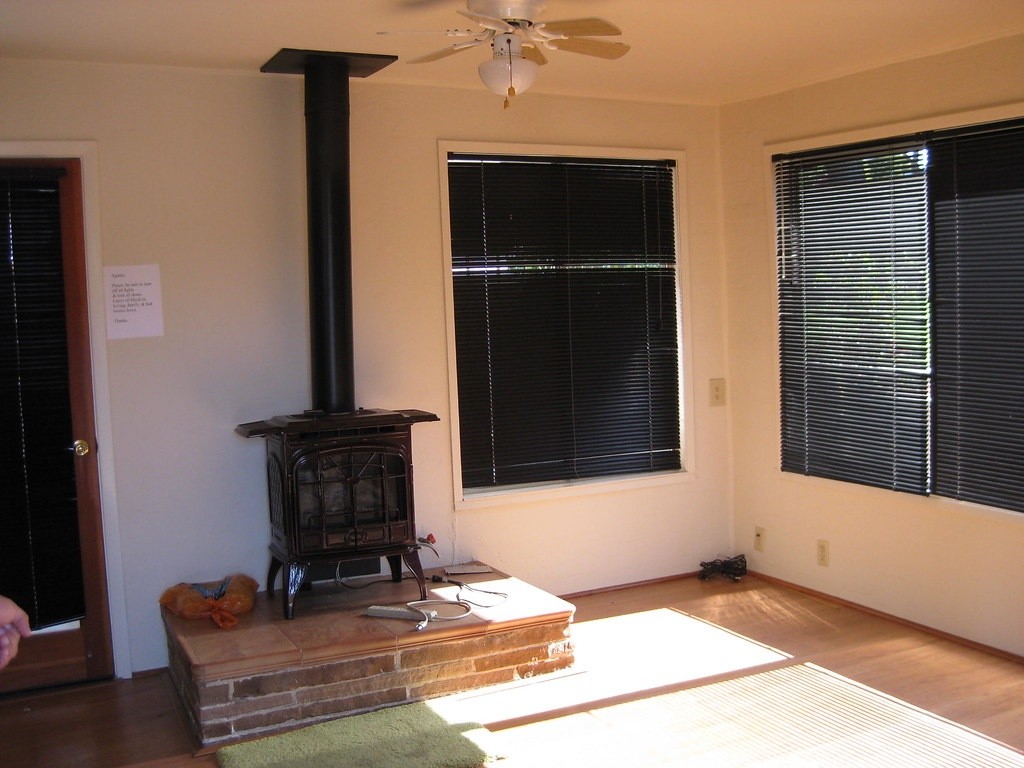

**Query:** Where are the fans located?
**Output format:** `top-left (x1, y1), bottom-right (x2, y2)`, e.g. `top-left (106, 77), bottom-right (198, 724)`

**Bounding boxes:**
top-left (376, 1), bottom-right (632, 111)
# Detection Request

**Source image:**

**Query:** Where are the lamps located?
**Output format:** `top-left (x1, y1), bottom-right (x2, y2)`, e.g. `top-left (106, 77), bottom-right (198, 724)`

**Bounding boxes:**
top-left (477, 33), bottom-right (540, 111)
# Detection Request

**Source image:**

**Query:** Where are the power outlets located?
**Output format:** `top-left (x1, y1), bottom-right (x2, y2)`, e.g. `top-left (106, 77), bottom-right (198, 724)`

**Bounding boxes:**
top-left (817, 539), bottom-right (829, 566)
top-left (709, 378), bottom-right (727, 406)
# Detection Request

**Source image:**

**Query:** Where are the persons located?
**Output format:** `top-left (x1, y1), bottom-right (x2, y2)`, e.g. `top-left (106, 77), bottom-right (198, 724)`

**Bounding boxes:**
top-left (0, 594), bottom-right (34, 671)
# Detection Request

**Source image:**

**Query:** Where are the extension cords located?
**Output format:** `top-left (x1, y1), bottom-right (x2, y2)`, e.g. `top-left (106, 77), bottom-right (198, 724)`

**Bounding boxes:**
top-left (367, 606), bottom-right (438, 621)
